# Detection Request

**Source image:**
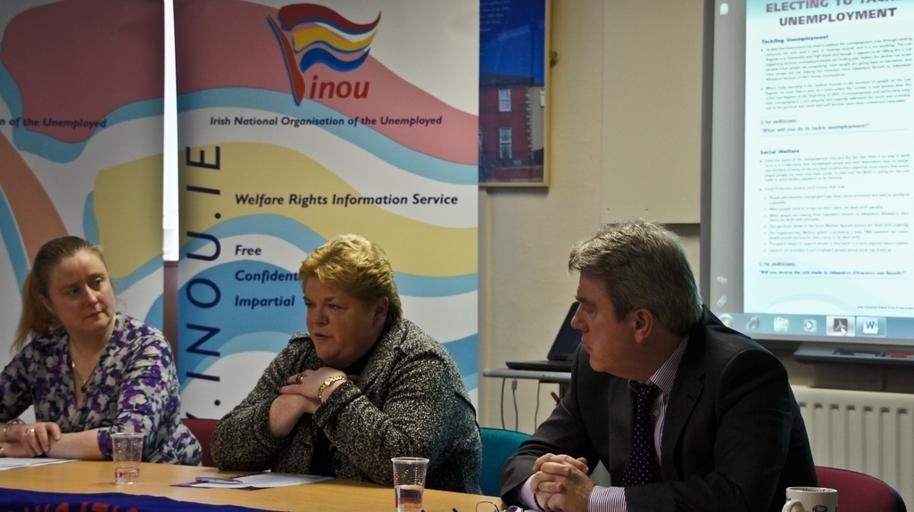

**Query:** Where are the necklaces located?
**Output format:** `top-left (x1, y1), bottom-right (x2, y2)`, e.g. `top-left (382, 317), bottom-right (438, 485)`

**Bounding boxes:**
top-left (66, 334), bottom-right (111, 393)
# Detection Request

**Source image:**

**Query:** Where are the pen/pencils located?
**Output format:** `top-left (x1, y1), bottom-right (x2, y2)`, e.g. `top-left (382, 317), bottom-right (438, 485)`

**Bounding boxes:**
top-left (195, 476), bottom-right (242, 485)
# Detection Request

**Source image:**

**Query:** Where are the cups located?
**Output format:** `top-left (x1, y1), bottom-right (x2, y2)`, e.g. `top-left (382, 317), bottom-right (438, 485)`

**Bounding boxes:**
top-left (107, 431), bottom-right (151, 488)
top-left (389, 455), bottom-right (432, 512)
top-left (775, 485), bottom-right (841, 512)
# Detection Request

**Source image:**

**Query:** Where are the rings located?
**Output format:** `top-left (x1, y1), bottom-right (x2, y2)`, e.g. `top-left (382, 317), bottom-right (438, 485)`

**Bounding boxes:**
top-left (295, 373), bottom-right (306, 385)
top-left (25, 428), bottom-right (35, 439)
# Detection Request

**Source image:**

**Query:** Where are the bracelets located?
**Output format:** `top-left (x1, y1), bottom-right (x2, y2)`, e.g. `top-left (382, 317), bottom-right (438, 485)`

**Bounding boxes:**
top-left (3, 417), bottom-right (26, 444)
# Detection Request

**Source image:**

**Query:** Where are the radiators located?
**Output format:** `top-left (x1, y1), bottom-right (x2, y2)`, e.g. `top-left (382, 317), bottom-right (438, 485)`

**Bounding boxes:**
top-left (788, 382), bottom-right (914, 512)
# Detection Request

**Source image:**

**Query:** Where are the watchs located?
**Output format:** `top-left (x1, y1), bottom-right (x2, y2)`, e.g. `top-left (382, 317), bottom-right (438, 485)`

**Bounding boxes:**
top-left (317, 374), bottom-right (347, 400)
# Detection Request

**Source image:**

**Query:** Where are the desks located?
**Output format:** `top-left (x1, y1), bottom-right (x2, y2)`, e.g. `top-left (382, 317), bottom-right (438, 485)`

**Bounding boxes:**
top-left (1, 457), bottom-right (505, 512)
top-left (482, 367), bottom-right (573, 406)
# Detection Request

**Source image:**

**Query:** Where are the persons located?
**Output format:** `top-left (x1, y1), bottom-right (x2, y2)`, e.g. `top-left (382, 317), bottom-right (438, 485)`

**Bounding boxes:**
top-left (500, 221), bottom-right (816, 512)
top-left (208, 234), bottom-right (483, 497)
top-left (0, 235), bottom-right (204, 466)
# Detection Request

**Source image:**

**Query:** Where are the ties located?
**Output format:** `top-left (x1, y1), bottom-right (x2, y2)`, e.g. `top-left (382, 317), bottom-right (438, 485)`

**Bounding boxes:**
top-left (622, 382), bottom-right (658, 487)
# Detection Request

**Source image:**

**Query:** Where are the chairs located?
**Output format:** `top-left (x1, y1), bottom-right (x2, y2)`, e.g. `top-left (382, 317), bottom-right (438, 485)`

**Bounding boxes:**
top-left (182, 415), bottom-right (908, 512)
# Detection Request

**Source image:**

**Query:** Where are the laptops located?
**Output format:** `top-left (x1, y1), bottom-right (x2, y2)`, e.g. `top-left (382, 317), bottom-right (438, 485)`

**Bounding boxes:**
top-left (505, 301), bottom-right (581, 372)
